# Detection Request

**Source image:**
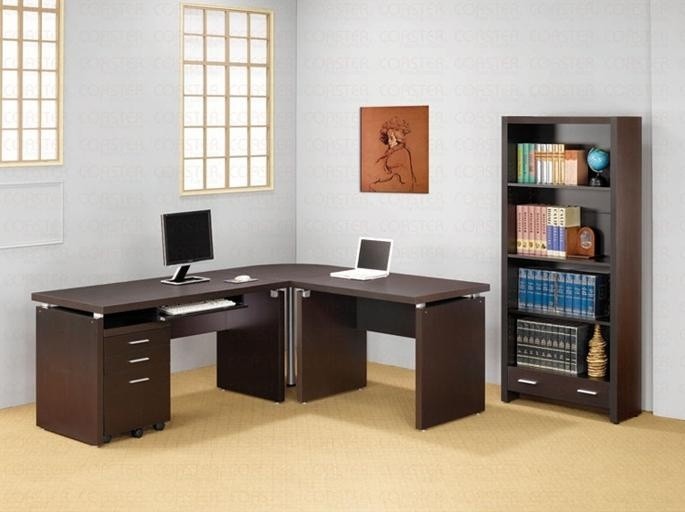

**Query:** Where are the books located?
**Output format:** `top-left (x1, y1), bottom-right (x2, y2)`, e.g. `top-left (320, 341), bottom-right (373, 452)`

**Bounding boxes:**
top-left (517, 264), bottom-right (610, 378)
top-left (517, 143), bottom-right (589, 185)
top-left (516, 203), bottom-right (581, 259)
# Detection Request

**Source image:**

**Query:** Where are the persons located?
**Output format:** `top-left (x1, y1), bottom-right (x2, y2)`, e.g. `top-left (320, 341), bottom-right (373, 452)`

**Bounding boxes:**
top-left (367, 116), bottom-right (418, 192)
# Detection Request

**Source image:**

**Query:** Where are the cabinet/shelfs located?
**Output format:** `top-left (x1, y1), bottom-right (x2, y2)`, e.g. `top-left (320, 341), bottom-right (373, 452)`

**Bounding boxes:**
top-left (501, 115), bottom-right (643, 425)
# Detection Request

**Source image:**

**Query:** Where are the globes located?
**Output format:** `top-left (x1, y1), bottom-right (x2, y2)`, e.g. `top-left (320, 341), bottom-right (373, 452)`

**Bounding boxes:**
top-left (587, 148), bottom-right (609, 187)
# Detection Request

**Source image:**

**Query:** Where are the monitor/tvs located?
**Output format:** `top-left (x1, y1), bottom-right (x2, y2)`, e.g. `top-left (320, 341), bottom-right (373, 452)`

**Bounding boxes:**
top-left (160, 208), bottom-right (215, 286)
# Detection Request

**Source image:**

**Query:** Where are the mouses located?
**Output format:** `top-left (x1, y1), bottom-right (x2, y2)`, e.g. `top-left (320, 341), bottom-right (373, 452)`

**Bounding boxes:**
top-left (235, 273), bottom-right (252, 282)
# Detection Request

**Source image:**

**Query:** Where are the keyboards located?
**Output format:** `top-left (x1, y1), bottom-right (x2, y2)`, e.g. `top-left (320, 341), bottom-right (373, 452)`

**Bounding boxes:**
top-left (162, 298), bottom-right (237, 316)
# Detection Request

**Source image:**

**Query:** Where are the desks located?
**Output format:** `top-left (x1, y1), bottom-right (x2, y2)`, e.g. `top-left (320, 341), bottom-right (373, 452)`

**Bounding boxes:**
top-left (32, 263), bottom-right (490, 446)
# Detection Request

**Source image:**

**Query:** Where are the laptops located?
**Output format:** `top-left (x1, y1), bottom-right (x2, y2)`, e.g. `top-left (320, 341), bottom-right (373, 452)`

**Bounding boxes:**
top-left (330, 237), bottom-right (394, 281)
